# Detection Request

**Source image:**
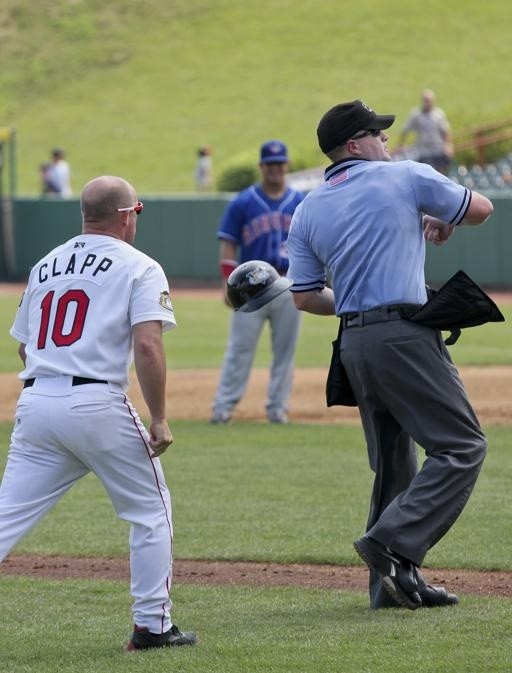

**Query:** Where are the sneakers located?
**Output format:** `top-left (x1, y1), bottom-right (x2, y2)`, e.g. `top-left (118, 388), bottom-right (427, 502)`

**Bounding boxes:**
top-left (353, 534), bottom-right (458, 610)
top-left (126, 623), bottom-right (199, 652)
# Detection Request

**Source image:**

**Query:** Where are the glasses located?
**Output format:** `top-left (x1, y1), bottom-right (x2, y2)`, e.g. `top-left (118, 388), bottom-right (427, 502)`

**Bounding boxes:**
top-left (116, 201), bottom-right (144, 215)
top-left (341, 129), bottom-right (381, 146)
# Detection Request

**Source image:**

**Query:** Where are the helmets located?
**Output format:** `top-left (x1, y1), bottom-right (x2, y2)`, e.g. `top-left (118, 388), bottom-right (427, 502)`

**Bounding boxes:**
top-left (226, 260), bottom-right (295, 313)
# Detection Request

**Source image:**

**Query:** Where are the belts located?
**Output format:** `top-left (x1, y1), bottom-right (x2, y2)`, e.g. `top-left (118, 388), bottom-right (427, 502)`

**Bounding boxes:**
top-left (23, 376), bottom-right (107, 388)
top-left (346, 309), bottom-right (401, 328)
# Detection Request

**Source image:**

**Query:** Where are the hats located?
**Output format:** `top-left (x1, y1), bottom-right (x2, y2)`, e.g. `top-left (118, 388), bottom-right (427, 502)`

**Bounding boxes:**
top-left (317, 100), bottom-right (396, 154)
top-left (261, 142), bottom-right (287, 164)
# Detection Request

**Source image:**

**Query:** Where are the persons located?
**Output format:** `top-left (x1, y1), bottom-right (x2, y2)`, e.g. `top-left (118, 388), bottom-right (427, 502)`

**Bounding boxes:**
top-left (1, 174), bottom-right (202, 654)
top-left (206, 140), bottom-right (315, 428)
top-left (195, 144), bottom-right (213, 192)
top-left (500, 150), bottom-right (512, 187)
top-left (287, 98), bottom-right (505, 613)
top-left (38, 147), bottom-right (72, 201)
top-left (389, 89), bottom-right (459, 201)
